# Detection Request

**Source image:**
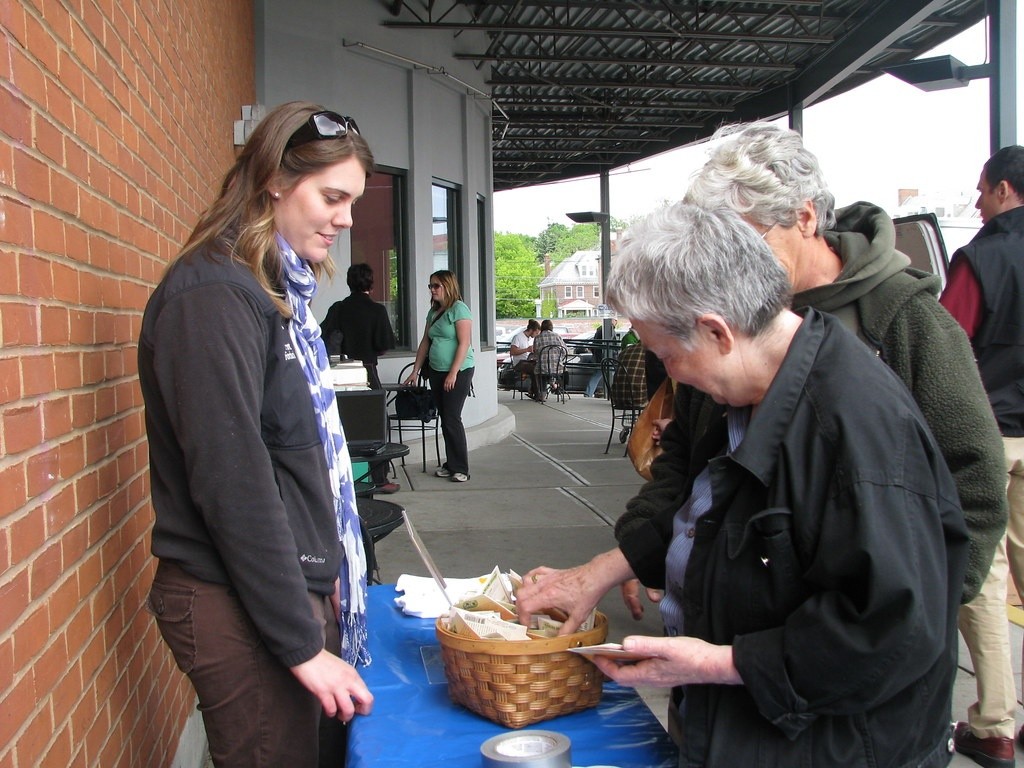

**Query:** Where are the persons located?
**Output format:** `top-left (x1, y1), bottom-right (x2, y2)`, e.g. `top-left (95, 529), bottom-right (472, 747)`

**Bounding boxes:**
top-left (620, 325), bottom-right (640, 350)
top-left (623, 121), bottom-right (1009, 623)
top-left (584, 318), bottom-right (618, 398)
top-left (138, 101), bottom-right (373, 768)
top-left (511, 319), bottom-right (568, 402)
top-left (610, 339), bottom-right (649, 407)
top-left (938, 145), bottom-right (1024, 768)
top-left (518, 205), bottom-right (960, 768)
top-left (403, 270), bottom-right (475, 482)
top-left (320, 263), bottom-right (401, 495)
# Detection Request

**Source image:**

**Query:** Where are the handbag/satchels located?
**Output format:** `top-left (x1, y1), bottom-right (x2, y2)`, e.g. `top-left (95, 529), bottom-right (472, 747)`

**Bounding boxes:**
top-left (393, 389), bottom-right (437, 418)
top-left (626, 378), bottom-right (675, 481)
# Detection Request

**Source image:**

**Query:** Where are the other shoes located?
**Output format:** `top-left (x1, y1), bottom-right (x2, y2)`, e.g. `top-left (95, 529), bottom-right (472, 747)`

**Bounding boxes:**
top-left (382, 483), bottom-right (400, 492)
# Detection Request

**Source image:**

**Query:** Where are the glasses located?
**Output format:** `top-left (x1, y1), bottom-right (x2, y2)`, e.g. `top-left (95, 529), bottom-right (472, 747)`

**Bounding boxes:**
top-left (284, 110), bottom-right (360, 149)
top-left (428, 283), bottom-right (444, 289)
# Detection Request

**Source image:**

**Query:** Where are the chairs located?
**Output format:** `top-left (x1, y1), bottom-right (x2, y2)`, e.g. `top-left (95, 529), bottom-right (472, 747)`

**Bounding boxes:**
top-left (510, 344), bottom-right (571, 404)
top-left (388, 362), bottom-right (441, 473)
top-left (601, 357), bottom-right (646, 457)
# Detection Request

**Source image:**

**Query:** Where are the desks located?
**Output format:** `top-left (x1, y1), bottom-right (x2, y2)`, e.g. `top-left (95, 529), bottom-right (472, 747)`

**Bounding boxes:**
top-left (347, 584), bottom-right (680, 768)
top-left (349, 442), bottom-right (410, 485)
top-left (355, 496), bottom-right (406, 542)
top-left (382, 382), bottom-right (413, 407)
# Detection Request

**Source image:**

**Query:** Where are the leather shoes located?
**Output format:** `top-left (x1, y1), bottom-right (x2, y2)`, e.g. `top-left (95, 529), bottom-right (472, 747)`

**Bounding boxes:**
top-left (955, 721), bottom-right (1015, 768)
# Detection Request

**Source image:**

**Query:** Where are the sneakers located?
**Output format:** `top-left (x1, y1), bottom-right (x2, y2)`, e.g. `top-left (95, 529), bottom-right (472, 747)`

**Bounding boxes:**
top-left (435, 468), bottom-right (451, 477)
top-left (451, 473), bottom-right (467, 482)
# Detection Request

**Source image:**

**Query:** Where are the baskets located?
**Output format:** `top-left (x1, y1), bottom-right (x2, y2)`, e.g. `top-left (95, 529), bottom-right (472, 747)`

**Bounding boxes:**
top-left (435, 611), bottom-right (608, 731)
